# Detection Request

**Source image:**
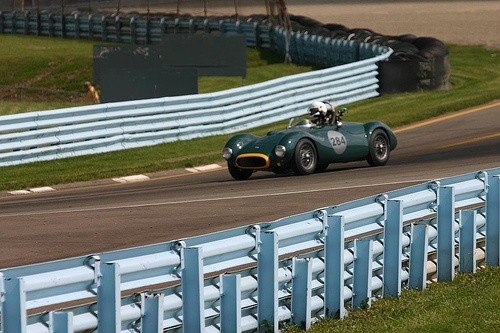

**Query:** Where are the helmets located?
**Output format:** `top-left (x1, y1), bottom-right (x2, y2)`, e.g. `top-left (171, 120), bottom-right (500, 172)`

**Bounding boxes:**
top-left (308, 101), bottom-right (327, 121)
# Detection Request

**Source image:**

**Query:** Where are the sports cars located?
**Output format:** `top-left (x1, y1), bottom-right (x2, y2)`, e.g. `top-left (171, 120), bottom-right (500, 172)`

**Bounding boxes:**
top-left (222, 99), bottom-right (398, 180)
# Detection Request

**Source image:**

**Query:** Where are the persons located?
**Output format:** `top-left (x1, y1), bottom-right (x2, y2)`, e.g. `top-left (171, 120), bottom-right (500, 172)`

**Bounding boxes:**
top-left (84, 82), bottom-right (100, 105)
top-left (307, 102), bottom-right (330, 126)
